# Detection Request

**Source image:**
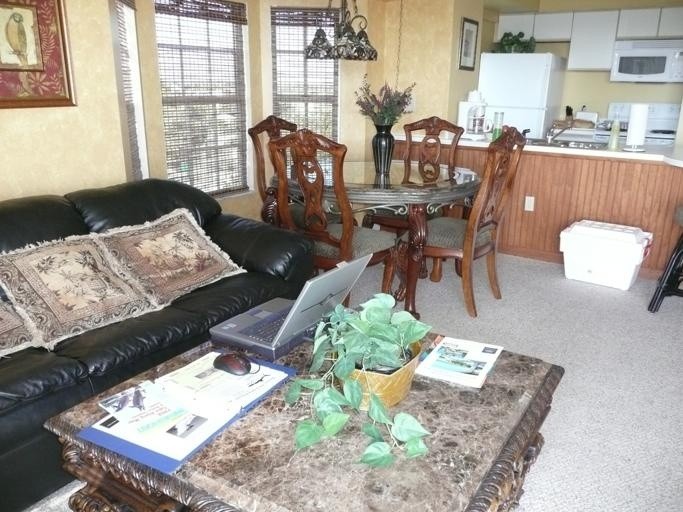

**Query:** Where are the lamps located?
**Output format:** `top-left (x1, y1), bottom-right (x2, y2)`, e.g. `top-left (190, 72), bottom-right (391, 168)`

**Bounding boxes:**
top-left (302, 2), bottom-right (377, 62)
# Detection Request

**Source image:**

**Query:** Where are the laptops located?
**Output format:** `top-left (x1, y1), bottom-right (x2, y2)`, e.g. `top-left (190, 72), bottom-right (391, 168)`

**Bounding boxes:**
top-left (208, 253), bottom-right (373, 359)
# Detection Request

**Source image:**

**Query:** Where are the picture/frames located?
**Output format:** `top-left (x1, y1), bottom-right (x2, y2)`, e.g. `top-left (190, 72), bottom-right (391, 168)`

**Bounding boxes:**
top-left (457, 16), bottom-right (480, 71)
top-left (0, 0), bottom-right (79, 110)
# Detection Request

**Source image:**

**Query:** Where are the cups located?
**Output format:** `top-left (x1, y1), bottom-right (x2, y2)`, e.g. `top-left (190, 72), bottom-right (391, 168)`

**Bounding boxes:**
top-left (492, 112), bottom-right (502, 140)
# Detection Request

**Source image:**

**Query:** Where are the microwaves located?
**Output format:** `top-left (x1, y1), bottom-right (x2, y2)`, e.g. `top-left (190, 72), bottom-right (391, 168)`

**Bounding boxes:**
top-left (608, 48), bottom-right (682, 83)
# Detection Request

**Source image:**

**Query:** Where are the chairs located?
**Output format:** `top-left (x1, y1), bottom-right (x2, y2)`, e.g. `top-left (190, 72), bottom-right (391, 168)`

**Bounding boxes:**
top-left (268, 129), bottom-right (398, 312)
top-left (248, 114), bottom-right (357, 225)
top-left (393, 126), bottom-right (528, 318)
top-left (363, 113), bottom-right (470, 229)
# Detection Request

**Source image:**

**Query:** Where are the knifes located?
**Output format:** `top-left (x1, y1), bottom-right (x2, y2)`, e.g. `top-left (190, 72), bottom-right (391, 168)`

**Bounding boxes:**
top-left (565, 105), bottom-right (573, 115)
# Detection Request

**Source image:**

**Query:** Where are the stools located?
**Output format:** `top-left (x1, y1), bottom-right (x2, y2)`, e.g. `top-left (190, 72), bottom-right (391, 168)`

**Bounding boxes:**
top-left (646, 234), bottom-right (683, 315)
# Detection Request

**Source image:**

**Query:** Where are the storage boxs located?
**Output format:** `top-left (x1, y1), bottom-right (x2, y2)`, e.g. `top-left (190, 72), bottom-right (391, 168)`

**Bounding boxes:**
top-left (559, 220), bottom-right (653, 292)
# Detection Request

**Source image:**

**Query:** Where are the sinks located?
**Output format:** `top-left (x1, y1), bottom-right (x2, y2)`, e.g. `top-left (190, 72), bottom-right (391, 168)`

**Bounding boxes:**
top-left (531, 136), bottom-right (563, 147)
top-left (563, 139), bottom-right (609, 152)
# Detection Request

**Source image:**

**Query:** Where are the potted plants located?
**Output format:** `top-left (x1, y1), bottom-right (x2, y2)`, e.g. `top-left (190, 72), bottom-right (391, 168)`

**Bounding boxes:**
top-left (292, 285), bottom-right (433, 462)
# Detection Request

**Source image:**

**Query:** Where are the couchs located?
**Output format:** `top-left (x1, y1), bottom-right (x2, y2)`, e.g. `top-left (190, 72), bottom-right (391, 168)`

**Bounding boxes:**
top-left (0, 174), bottom-right (316, 510)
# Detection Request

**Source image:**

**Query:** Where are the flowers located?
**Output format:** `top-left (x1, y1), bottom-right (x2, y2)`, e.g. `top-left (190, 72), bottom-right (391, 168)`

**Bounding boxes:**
top-left (354, 71), bottom-right (418, 127)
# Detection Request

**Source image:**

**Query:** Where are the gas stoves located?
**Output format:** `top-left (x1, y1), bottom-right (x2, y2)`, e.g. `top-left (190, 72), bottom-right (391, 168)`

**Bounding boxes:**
top-left (594, 129), bottom-right (677, 140)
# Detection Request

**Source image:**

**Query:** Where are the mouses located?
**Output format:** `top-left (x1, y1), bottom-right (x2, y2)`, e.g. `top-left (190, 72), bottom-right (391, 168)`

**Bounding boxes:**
top-left (213, 353), bottom-right (251, 376)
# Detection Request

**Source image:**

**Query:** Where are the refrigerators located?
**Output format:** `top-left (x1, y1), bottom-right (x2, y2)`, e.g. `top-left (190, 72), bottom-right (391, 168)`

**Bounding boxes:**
top-left (476, 51), bottom-right (568, 142)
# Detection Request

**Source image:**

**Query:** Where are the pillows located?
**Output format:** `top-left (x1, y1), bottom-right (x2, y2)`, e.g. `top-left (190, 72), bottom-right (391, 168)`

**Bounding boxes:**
top-left (1, 234), bottom-right (161, 348)
top-left (85, 207), bottom-right (246, 307)
top-left (0, 279), bottom-right (41, 358)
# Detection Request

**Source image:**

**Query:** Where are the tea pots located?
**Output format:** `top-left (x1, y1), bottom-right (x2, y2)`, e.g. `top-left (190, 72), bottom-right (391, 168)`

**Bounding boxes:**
top-left (457, 100), bottom-right (492, 141)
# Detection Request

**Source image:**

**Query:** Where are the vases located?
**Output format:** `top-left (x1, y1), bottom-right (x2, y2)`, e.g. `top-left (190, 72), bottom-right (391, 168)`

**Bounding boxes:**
top-left (368, 124), bottom-right (397, 175)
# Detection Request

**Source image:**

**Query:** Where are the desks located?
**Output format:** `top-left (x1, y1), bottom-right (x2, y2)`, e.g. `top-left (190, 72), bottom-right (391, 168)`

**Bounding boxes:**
top-left (270, 157), bottom-right (483, 320)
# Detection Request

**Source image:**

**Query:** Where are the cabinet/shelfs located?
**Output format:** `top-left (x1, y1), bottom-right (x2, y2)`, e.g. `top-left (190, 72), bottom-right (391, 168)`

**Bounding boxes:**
top-left (491, 11), bottom-right (574, 43)
top-left (566, 12), bottom-right (620, 72)
top-left (616, 6), bottom-right (682, 40)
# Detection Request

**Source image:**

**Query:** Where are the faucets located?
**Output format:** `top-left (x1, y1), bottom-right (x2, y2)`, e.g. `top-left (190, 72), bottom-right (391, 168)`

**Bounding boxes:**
top-left (545, 105), bottom-right (575, 145)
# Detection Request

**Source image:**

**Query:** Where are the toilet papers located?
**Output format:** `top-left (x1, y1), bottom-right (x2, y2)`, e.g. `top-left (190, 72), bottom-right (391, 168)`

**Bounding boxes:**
top-left (626, 102), bottom-right (649, 146)
